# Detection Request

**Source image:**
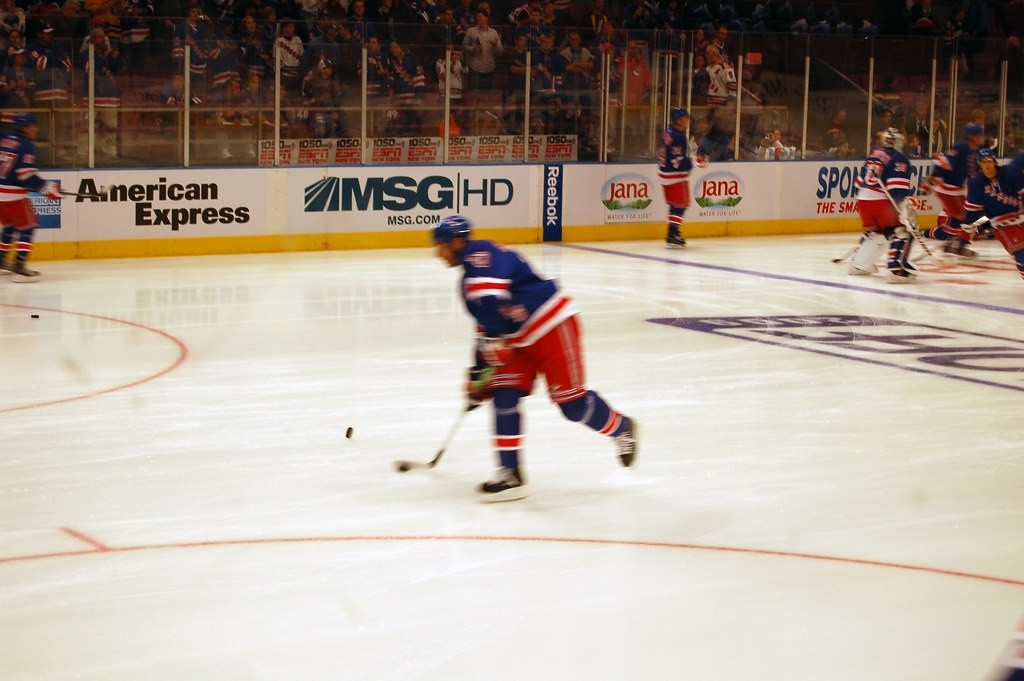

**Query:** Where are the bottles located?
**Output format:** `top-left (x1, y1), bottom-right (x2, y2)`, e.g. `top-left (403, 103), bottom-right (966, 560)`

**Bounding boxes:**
top-left (773, 138), bottom-right (783, 160)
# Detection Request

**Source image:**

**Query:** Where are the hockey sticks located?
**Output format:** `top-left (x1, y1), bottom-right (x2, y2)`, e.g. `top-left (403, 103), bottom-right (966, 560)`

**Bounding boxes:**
top-left (31, 315), bottom-right (39, 318)
top-left (26, 184), bottom-right (108, 198)
top-left (871, 168), bottom-right (958, 267)
top-left (832, 230), bottom-right (870, 263)
top-left (936, 215), bottom-right (1024, 236)
top-left (392, 364), bottom-right (499, 470)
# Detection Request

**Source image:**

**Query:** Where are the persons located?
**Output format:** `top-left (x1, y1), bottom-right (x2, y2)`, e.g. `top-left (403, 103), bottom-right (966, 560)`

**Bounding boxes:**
top-left (658, 108), bottom-right (696, 247)
top-left (918, 122), bottom-right (997, 241)
top-left (429, 216), bottom-right (636, 494)
top-left (0, 0), bottom-right (1024, 165)
top-left (982, 613), bottom-right (1024, 681)
top-left (0, 112), bottom-right (66, 278)
top-left (962, 148), bottom-right (1024, 280)
top-left (853, 128), bottom-right (918, 278)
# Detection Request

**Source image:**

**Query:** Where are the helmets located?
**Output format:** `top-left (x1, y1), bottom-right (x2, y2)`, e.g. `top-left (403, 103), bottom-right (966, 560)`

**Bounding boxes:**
top-left (882, 129), bottom-right (904, 149)
top-left (431, 215), bottom-right (471, 242)
top-left (13, 112), bottom-right (36, 127)
top-left (975, 147), bottom-right (995, 163)
top-left (670, 107), bottom-right (688, 118)
top-left (964, 122), bottom-right (983, 134)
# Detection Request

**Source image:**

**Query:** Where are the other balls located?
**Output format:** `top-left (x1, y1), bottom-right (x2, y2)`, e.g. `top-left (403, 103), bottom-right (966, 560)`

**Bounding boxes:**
top-left (346, 427), bottom-right (354, 438)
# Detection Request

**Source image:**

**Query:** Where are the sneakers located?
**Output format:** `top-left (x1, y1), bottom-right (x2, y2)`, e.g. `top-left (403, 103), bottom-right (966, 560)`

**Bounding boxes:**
top-left (944, 240), bottom-right (976, 258)
top-left (10, 263), bottom-right (41, 282)
top-left (0, 265), bottom-right (13, 276)
top-left (890, 268), bottom-right (918, 282)
top-left (903, 259), bottom-right (920, 272)
top-left (479, 466), bottom-right (529, 503)
top-left (617, 419), bottom-right (642, 466)
top-left (666, 234), bottom-right (687, 249)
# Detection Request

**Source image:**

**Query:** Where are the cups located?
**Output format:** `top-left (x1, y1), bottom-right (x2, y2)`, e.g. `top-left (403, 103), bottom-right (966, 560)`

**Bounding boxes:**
top-left (765, 145), bottom-right (797, 160)
